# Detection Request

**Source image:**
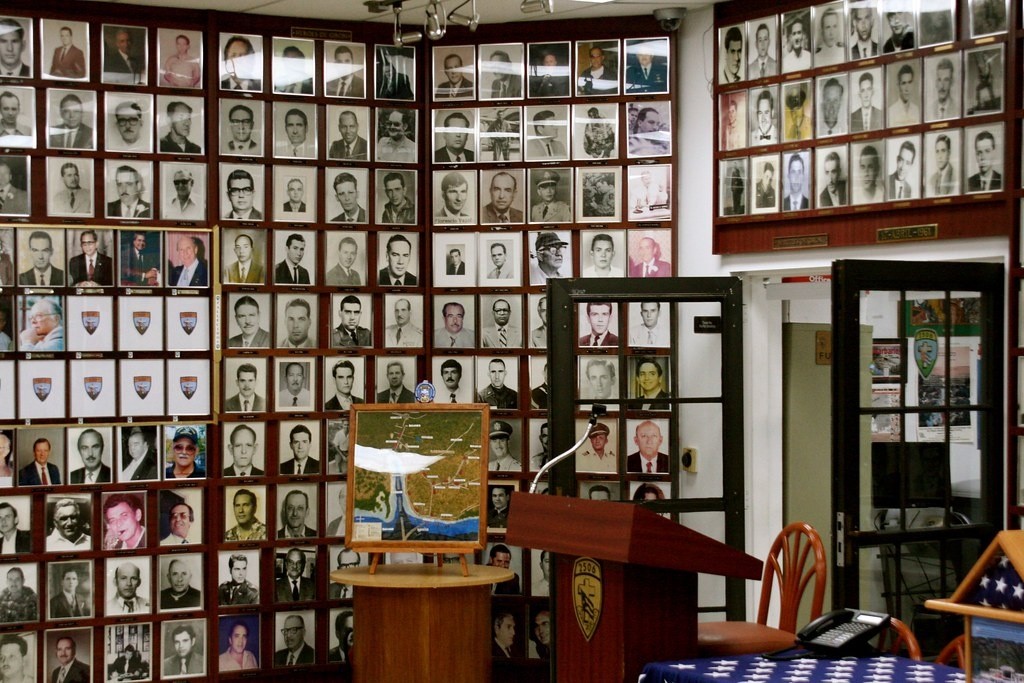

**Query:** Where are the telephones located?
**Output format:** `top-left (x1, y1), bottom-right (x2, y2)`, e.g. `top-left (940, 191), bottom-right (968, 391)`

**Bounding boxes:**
top-left (795, 607), bottom-right (892, 657)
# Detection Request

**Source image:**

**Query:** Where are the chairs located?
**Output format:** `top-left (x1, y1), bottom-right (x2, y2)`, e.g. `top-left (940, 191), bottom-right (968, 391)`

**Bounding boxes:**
top-left (695, 522), bottom-right (828, 659)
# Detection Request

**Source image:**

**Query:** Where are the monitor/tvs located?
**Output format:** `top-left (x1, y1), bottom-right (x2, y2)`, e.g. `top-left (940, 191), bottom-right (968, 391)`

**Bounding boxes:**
top-left (870, 441), bottom-right (953, 508)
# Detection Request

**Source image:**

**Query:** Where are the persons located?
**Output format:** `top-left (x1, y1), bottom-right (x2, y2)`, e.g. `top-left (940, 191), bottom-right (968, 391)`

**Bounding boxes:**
top-left (719, 0), bottom-right (1002, 213)
top-left (0, 17), bottom-right (671, 683)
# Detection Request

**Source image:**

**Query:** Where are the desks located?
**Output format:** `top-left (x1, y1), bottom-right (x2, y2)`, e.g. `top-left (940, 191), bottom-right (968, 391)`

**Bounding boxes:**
top-left (330, 564), bottom-right (515, 683)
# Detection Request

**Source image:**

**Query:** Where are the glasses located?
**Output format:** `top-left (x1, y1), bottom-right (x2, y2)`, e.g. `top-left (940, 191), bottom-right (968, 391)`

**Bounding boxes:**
top-left (82, 241), bottom-right (96, 246)
top-left (174, 444), bottom-right (198, 456)
top-left (541, 245), bottom-right (565, 254)
top-left (117, 118), bottom-right (140, 128)
top-left (231, 187), bottom-right (252, 196)
top-left (174, 180), bottom-right (191, 185)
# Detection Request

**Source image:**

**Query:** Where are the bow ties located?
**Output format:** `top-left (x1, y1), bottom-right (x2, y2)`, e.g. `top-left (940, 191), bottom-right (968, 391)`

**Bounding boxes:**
top-left (760, 135), bottom-right (770, 140)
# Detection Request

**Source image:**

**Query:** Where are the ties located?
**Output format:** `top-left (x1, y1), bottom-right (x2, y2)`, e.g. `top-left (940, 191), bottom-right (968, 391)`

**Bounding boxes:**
top-left (938, 174), bottom-right (941, 194)
top-left (0, 13), bottom-right (677, 683)
top-left (792, 201), bottom-right (797, 210)
top-left (828, 130), bottom-right (831, 135)
top-left (863, 48), bottom-right (867, 58)
top-left (982, 180), bottom-right (986, 191)
top-left (865, 113), bottom-right (868, 130)
top-left (940, 108), bottom-right (945, 120)
top-left (898, 186), bottom-right (902, 198)
top-left (760, 62), bottom-right (765, 78)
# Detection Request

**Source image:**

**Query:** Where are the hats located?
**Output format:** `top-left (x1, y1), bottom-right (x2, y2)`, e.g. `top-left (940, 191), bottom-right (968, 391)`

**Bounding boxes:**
top-left (589, 423), bottom-right (610, 438)
top-left (535, 169), bottom-right (560, 187)
top-left (172, 427), bottom-right (198, 444)
top-left (534, 233), bottom-right (569, 250)
top-left (489, 421), bottom-right (513, 440)
top-left (785, 88), bottom-right (806, 109)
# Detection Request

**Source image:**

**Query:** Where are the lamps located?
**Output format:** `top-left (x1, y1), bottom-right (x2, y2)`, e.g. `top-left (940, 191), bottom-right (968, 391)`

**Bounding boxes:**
top-left (362, 0), bottom-right (553, 49)
